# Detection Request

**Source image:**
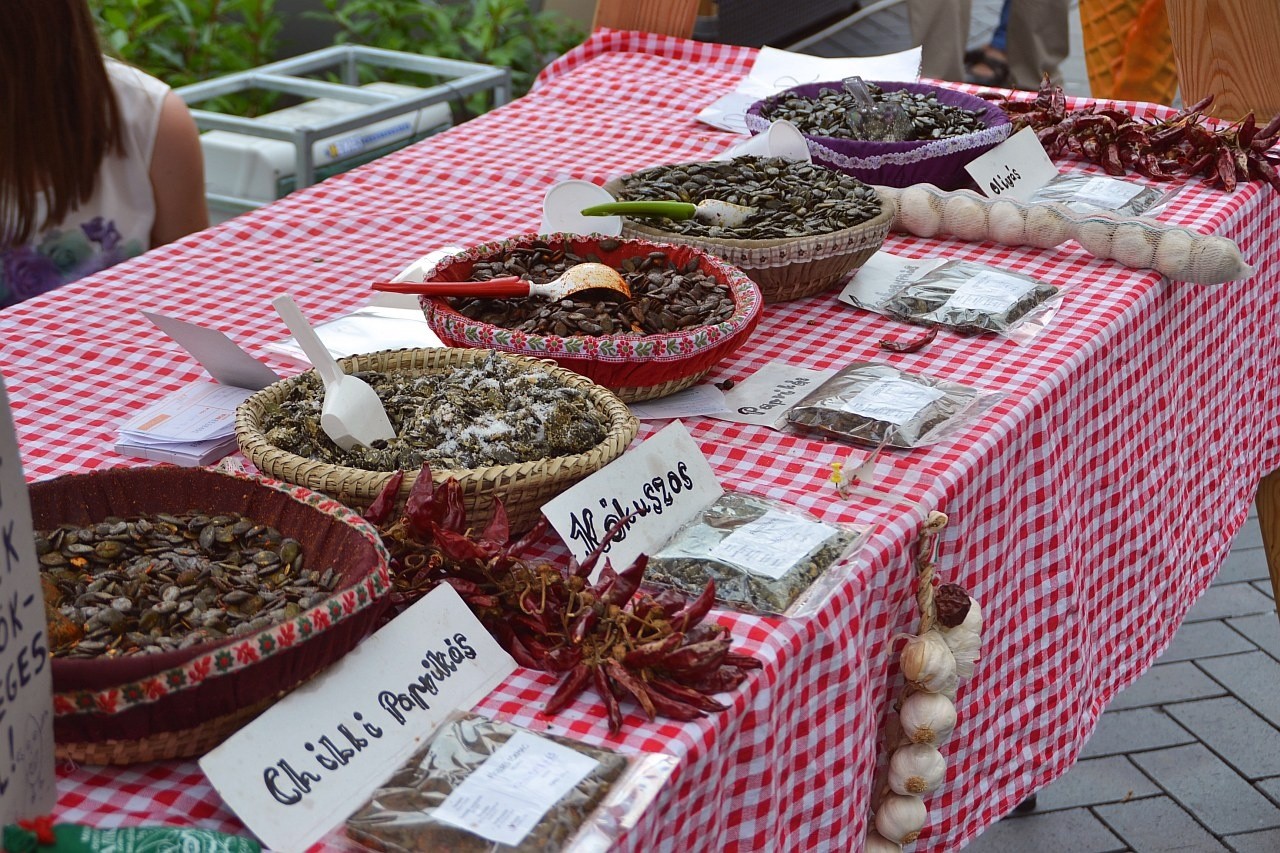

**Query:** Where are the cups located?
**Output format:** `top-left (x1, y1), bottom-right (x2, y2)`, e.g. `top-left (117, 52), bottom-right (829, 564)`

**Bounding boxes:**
top-left (707, 119), bottom-right (813, 165)
top-left (539, 179), bottom-right (625, 239)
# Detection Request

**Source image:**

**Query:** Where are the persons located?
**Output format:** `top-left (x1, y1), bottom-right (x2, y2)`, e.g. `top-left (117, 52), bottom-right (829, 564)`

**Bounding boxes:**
top-left (907, 0), bottom-right (1071, 94)
top-left (961, 0), bottom-right (1011, 86)
top-left (0, 0), bottom-right (212, 312)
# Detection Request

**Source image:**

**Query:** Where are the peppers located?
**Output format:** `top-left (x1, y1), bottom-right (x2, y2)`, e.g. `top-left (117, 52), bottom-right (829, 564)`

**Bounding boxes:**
top-left (975, 71), bottom-right (1279, 194)
top-left (361, 462), bottom-right (766, 740)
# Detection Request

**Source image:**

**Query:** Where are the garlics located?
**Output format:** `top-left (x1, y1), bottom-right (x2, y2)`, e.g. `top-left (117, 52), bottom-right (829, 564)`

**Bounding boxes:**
top-left (862, 184), bottom-right (1258, 284)
top-left (862, 583), bottom-right (983, 853)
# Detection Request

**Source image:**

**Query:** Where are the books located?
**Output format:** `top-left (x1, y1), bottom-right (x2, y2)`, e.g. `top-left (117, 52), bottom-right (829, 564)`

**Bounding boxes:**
top-left (114, 311), bottom-right (281, 466)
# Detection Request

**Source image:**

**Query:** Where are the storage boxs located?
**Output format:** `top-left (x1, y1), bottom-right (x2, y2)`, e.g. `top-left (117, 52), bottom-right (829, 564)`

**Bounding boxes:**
top-left (1, 36), bottom-right (1280, 853)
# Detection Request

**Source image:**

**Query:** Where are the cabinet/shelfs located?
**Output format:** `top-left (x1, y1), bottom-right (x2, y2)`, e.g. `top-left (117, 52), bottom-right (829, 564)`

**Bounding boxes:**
top-left (167, 45), bottom-right (511, 210)
